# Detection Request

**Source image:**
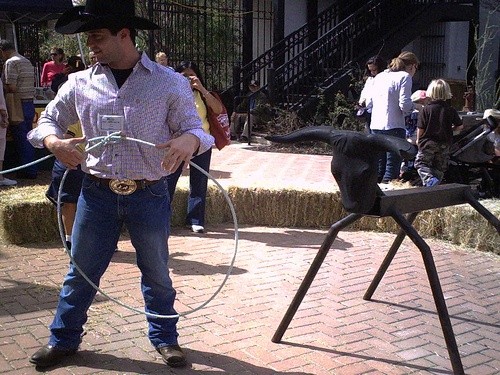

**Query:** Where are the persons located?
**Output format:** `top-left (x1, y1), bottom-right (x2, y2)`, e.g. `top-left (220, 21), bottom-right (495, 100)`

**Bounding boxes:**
top-left (45, 83), bottom-right (118, 252)
top-left (155, 52), bottom-right (174, 71)
top-left (27, 0), bottom-right (214, 366)
top-left (167, 61), bottom-right (223, 233)
top-left (354, 51), bottom-right (463, 188)
top-left (463, 108), bottom-right (500, 200)
top-left (0, 40), bottom-right (37, 186)
top-left (40, 48), bottom-right (99, 86)
top-left (228, 80), bottom-right (267, 143)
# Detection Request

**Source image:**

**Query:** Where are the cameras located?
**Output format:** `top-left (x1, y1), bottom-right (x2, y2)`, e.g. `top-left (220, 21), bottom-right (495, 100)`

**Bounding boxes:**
top-left (185, 77), bottom-right (193, 92)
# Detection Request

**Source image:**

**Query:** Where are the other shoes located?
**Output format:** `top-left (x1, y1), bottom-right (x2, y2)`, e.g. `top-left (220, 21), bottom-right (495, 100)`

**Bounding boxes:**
top-left (230, 136), bottom-right (236, 140)
top-left (425, 177), bottom-right (439, 186)
top-left (240, 137), bottom-right (247, 141)
top-left (185, 223), bottom-right (205, 232)
top-left (0, 178), bottom-right (17, 186)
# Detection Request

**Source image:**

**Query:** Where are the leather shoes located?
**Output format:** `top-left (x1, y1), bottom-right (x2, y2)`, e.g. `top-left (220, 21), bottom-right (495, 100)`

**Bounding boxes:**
top-left (29, 343), bottom-right (76, 365)
top-left (159, 345), bottom-right (186, 367)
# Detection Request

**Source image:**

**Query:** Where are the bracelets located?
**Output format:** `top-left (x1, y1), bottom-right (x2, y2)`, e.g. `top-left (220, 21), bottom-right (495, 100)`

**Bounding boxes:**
top-left (203, 91), bottom-right (209, 98)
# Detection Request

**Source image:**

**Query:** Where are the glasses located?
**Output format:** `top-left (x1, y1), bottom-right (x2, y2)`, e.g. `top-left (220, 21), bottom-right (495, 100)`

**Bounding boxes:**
top-left (91, 57), bottom-right (95, 59)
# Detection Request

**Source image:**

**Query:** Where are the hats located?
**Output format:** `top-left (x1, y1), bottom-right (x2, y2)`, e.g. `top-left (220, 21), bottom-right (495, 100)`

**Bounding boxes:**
top-left (56, 0), bottom-right (162, 35)
top-left (50, 48), bottom-right (59, 54)
top-left (249, 80), bottom-right (259, 86)
top-left (482, 108), bottom-right (500, 120)
top-left (411, 89), bottom-right (427, 102)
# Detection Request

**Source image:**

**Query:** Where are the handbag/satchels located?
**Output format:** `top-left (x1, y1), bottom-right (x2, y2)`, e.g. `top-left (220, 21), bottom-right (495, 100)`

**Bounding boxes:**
top-left (200, 90), bottom-right (231, 150)
top-left (5, 93), bottom-right (24, 122)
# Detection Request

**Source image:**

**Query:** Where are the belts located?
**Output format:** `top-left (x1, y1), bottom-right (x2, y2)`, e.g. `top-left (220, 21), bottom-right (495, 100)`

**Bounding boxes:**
top-left (97, 177), bottom-right (150, 195)
